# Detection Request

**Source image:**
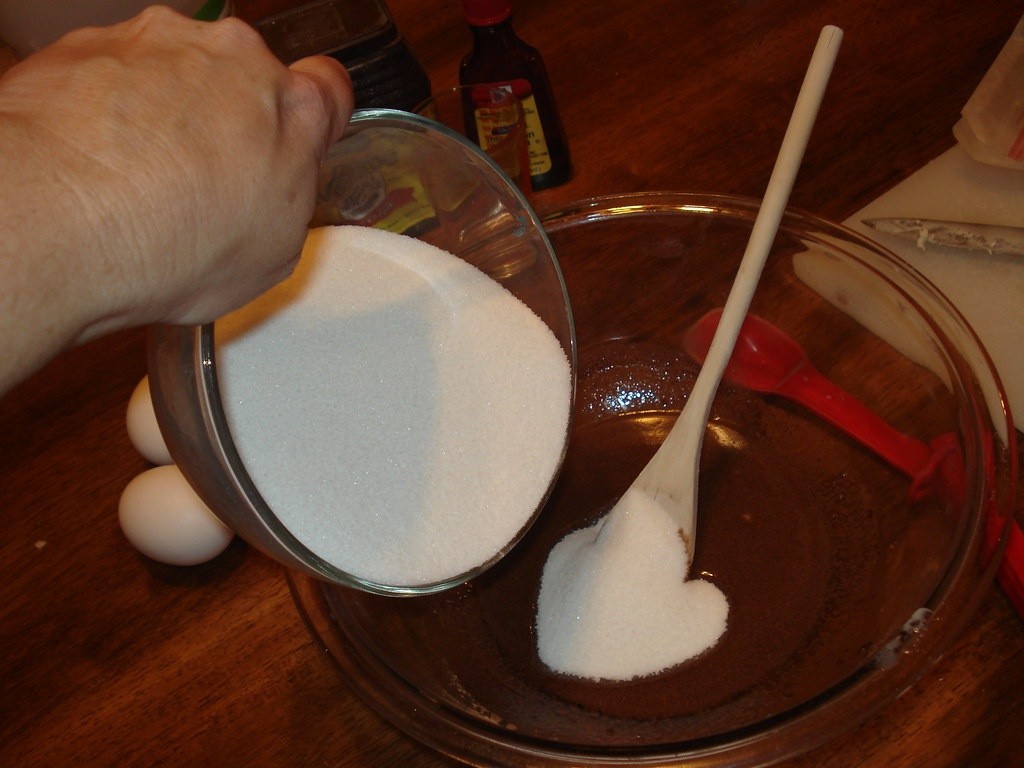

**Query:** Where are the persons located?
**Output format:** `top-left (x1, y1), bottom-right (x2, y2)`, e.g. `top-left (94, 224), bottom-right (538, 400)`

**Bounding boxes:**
top-left (0, 4), bottom-right (355, 401)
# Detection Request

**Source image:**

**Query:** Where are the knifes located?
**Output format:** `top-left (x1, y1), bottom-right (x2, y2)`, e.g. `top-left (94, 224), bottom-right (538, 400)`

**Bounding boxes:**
top-left (860, 210), bottom-right (1024, 262)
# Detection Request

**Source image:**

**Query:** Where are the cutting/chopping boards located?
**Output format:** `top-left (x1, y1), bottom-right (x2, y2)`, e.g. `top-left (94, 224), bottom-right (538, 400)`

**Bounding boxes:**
top-left (790, 138), bottom-right (1024, 434)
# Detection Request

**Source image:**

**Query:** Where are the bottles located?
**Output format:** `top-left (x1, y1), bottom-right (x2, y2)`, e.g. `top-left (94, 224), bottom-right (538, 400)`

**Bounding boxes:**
top-left (451, 1), bottom-right (574, 192)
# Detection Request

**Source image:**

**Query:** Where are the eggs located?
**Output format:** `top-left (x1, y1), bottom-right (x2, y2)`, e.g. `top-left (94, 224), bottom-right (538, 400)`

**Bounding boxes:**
top-left (117, 463), bottom-right (234, 566)
top-left (125, 373), bottom-right (176, 463)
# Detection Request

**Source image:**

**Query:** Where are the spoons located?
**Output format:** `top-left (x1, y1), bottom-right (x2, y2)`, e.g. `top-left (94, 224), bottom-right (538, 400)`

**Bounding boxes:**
top-left (596, 23), bottom-right (844, 584)
top-left (679, 300), bottom-right (980, 500)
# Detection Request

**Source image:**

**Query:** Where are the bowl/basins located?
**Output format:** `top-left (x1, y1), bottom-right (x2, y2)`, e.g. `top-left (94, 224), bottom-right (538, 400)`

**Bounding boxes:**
top-left (286, 192), bottom-right (1012, 764)
top-left (142, 102), bottom-right (576, 601)
